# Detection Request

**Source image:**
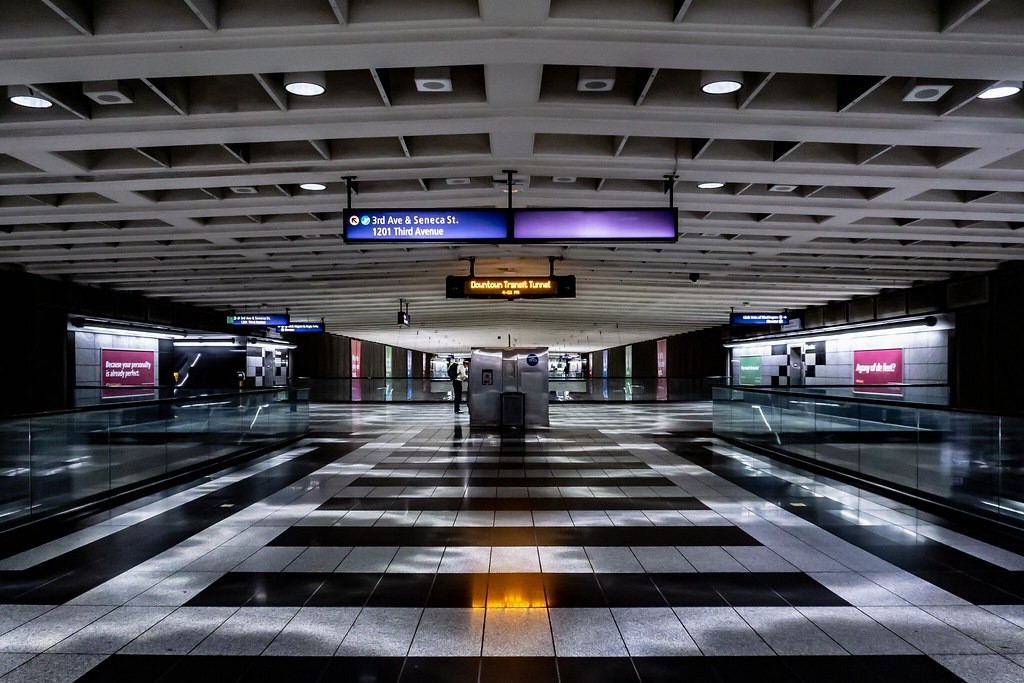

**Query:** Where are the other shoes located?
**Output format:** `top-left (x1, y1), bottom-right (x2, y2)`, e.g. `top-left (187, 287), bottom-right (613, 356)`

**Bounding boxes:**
top-left (455, 410), bottom-right (464, 413)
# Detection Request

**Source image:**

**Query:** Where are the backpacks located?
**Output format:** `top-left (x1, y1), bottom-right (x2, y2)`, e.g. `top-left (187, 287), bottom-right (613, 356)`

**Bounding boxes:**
top-left (448, 363), bottom-right (461, 381)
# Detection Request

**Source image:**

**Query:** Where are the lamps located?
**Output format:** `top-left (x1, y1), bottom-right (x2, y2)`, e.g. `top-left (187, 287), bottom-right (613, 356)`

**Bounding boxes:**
top-left (282, 71), bottom-right (327, 97)
top-left (698, 182), bottom-right (725, 189)
top-left (8, 85), bottom-right (52, 108)
top-left (299, 183), bottom-right (327, 190)
top-left (699, 70), bottom-right (743, 95)
top-left (978, 81), bottom-right (1023, 99)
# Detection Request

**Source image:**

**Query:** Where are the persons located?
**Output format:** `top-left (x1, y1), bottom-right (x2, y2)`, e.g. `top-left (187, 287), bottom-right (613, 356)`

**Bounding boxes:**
top-left (553, 366), bottom-right (569, 377)
top-left (453, 360), bottom-right (469, 413)
